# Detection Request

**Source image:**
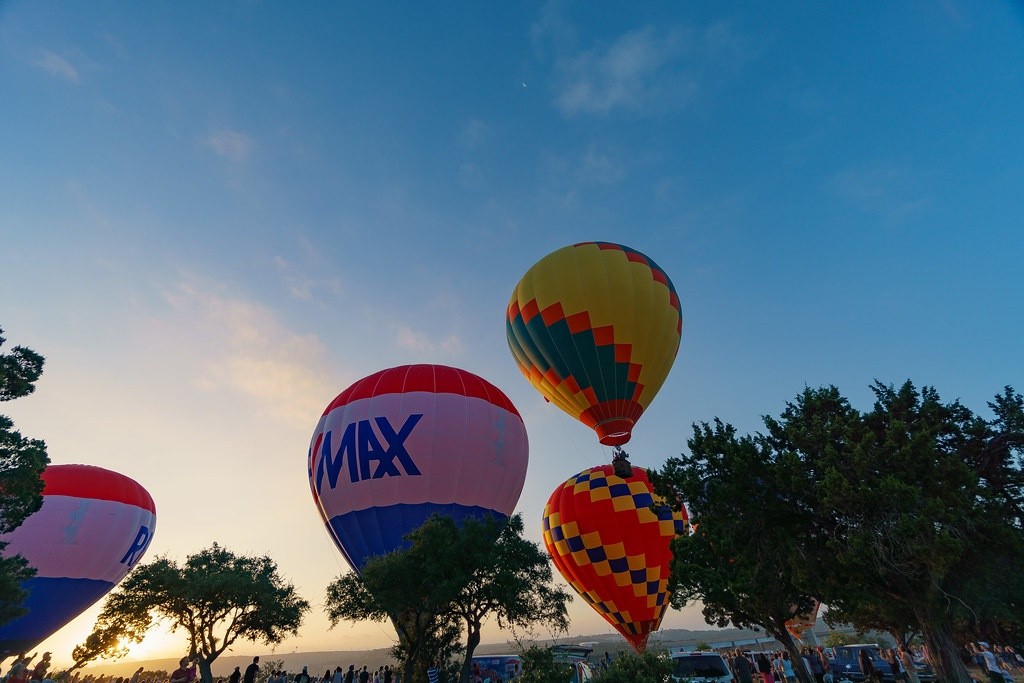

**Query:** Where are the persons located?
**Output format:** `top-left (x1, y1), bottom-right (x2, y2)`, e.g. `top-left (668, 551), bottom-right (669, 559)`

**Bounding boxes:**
top-left (0, 652), bottom-right (466, 683)
top-left (600, 639), bottom-right (1023, 683)
top-left (614, 450), bottom-right (629, 462)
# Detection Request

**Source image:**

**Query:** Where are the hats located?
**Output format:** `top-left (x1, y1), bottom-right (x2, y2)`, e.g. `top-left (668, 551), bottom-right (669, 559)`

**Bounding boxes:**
top-left (978, 641), bottom-right (990, 649)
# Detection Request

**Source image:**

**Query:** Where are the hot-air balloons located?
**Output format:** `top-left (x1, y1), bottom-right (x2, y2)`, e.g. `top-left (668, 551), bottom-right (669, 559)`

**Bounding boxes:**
top-left (540, 463), bottom-right (689, 656)
top-left (0, 464), bottom-right (156, 656)
top-left (689, 507), bottom-right (824, 646)
top-left (503, 240), bottom-right (681, 477)
top-left (307, 362), bottom-right (528, 645)
